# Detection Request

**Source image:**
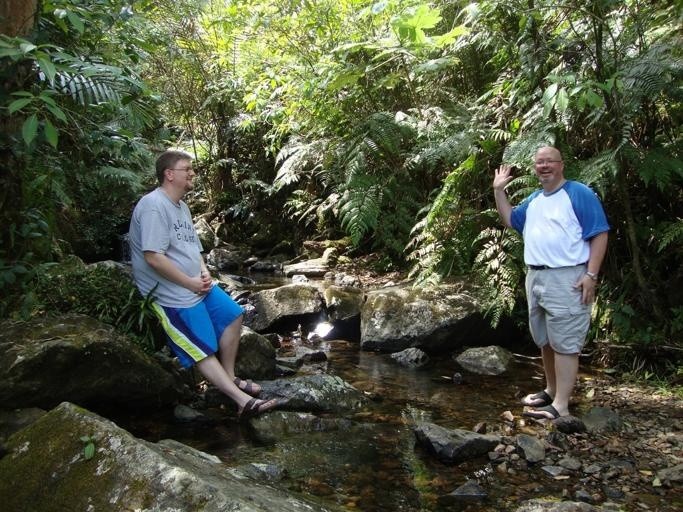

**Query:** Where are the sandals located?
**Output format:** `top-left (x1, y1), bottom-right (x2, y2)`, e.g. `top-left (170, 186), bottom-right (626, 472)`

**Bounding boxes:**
top-left (521, 389), bottom-right (554, 407)
top-left (233, 375), bottom-right (262, 397)
top-left (525, 403), bottom-right (561, 419)
top-left (234, 397), bottom-right (279, 424)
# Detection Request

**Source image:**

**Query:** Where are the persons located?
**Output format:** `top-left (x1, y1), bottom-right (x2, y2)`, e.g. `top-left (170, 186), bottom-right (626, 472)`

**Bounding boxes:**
top-left (128, 152), bottom-right (278, 420)
top-left (491, 146), bottom-right (612, 420)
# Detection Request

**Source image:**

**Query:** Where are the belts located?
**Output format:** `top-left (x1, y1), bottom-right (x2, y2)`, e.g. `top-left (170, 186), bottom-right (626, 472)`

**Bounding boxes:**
top-left (527, 262), bottom-right (588, 270)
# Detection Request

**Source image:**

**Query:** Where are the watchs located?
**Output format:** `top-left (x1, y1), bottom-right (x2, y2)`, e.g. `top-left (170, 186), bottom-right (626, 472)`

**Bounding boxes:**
top-left (586, 271), bottom-right (598, 281)
